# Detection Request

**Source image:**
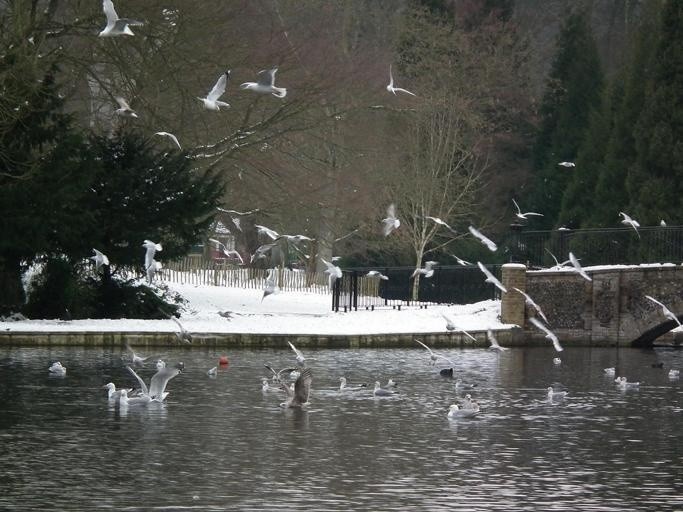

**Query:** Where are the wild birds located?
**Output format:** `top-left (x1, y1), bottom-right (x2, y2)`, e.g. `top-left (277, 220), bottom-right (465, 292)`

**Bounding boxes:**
top-left (196, 69), bottom-right (231, 111)
top-left (319, 200), bottom-right (681, 420)
top-left (207, 206), bottom-right (317, 304)
top-left (259, 340), bottom-right (318, 411)
top-left (102, 314), bottom-right (218, 408)
top-left (386, 64), bottom-right (417, 98)
top-left (114, 95), bottom-right (138, 120)
top-left (90, 249), bottom-right (110, 270)
top-left (154, 131), bottom-right (182, 153)
top-left (98, 0), bottom-right (144, 36)
top-left (557, 161), bottom-right (576, 169)
top-left (48, 361), bottom-right (67, 375)
top-left (240, 68), bottom-right (287, 98)
